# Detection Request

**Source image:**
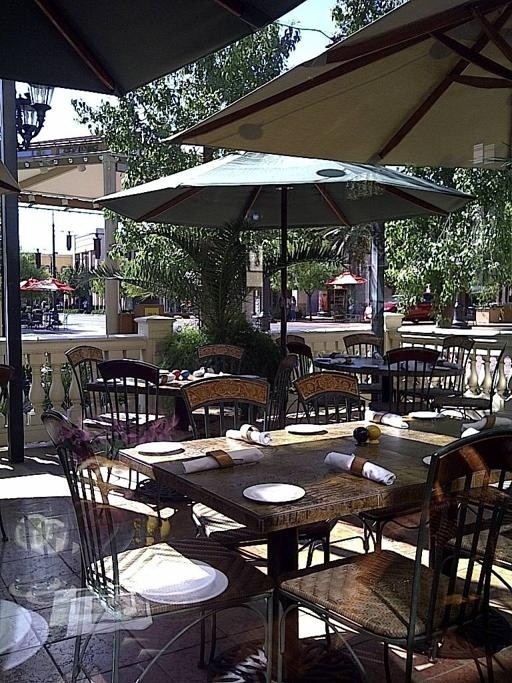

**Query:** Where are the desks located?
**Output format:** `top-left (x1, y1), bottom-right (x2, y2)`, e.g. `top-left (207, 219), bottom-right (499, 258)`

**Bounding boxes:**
top-left (116, 419), bottom-right (509, 683)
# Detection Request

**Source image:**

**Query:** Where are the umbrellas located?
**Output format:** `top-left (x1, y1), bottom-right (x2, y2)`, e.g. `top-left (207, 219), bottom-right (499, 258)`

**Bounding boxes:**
top-left (19, 276), bottom-right (40, 307)
top-left (91, 150), bottom-right (480, 356)
top-left (325, 271), bottom-right (368, 314)
top-left (0, 0), bottom-right (307, 466)
top-left (158, 0), bottom-right (511, 172)
top-left (27, 276), bottom-right (75, 305)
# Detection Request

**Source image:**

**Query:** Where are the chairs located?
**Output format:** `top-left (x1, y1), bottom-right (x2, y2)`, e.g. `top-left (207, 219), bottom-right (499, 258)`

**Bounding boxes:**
top-left (20, 300), bottom-right (60, 329)
top-left (264, 424), bottom-right (511, 681)
top-left (39, 401), bottom-right (276, 683)
top-left (39, 333), bottom-right (511, 520)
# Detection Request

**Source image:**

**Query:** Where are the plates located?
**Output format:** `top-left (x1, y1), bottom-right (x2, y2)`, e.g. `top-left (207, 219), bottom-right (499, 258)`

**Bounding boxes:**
top-left (135, 441), bottom-right (182, 454)
top-left (430, 365), bottom-right (451, 370)
top-left (285, 423), bottom-right (324, 434)
top-left (97, 378), bottom-right (121, 383)
top-left (408, 411), bottom-right (442, 418)
top-left (317, 358), bottom-right (331, 361)
top-left (242, 483), bottom-right (307, 503)
top-left (422, 456), bottom-right (432, 465)
top-left (0, 599), bottom-right (50, 672)
top-left (129, 558), bottom-right (229, 605)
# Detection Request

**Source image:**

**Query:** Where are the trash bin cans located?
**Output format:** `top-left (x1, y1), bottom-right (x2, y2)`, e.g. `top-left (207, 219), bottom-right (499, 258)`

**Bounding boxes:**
top-left (118, 308), bottom-right (135, 334)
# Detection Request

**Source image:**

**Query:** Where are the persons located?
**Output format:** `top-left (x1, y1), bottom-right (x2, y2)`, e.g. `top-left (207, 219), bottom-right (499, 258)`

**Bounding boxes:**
top-left (288, 296), bottom-right (297, 322)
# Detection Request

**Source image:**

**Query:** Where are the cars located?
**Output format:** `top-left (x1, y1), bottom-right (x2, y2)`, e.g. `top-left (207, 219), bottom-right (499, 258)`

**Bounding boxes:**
top-left (362, 290), bottom-right (439, 327)
